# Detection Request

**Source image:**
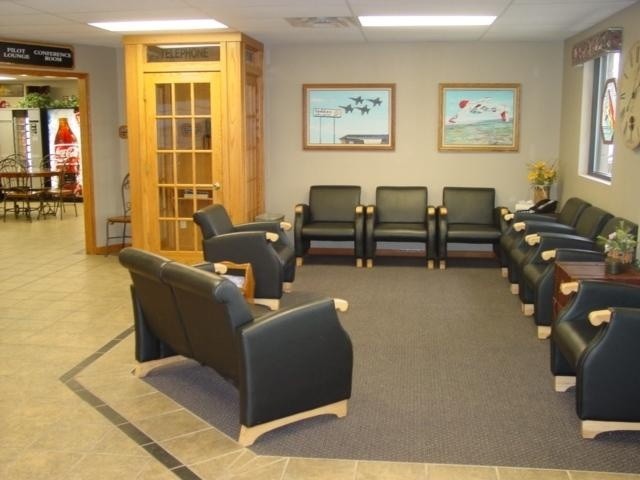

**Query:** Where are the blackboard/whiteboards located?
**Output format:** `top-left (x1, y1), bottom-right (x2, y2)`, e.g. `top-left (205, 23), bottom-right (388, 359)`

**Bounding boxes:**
top-left (0, 40), bottom-right (75, 70)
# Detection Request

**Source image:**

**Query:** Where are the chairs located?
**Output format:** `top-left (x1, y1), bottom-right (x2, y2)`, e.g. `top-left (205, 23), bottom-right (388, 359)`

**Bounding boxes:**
top-left (292, 184), bottom-right (365, 272)
top-left (433, 184), bottom-right (511, 271)
top-left (0, 154), bottom-right (82, 221)
top-left (101, 170), bottom-right (135, 263)
top-left (368, 185), bottom-right (438, 272)
top-left (500, 192), bottom-right (636, 339)
top-left (545, 279), bottom-right (640, 443)
top-left (190, 203), bottom-right (299, 314)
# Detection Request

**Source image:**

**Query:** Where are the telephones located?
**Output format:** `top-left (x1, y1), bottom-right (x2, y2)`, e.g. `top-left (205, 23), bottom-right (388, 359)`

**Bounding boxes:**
top-left (534, 198), bottom-right (557, 213)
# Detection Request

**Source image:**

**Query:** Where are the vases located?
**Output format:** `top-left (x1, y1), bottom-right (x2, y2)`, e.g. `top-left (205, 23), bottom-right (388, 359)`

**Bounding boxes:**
top-left (532, 185), bottom-right (552, 207)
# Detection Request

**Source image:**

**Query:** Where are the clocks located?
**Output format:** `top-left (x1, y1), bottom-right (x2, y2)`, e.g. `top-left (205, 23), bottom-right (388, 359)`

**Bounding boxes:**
top-left (614, 40), bottom-right (640, 151)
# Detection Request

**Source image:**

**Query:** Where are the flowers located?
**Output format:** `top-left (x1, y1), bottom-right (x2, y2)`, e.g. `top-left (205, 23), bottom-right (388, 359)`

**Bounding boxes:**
top-left (525, 158), bottom-right (563, 186)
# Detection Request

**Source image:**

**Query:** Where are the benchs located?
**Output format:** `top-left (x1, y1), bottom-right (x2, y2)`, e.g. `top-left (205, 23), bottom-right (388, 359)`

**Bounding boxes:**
top-left (115, 240), bottom-right (355, 451)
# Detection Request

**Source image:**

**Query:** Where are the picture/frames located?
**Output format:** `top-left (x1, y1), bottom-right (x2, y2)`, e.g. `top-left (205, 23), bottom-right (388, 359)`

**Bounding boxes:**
top-left (436, 81), bottom-right (523, 155)
top-left (299, 81), bottom-right (397, 153)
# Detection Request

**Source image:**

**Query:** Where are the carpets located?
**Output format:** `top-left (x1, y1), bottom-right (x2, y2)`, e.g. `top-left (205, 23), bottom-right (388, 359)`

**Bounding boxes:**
top-left (132, 249), bottom-right (639, 475)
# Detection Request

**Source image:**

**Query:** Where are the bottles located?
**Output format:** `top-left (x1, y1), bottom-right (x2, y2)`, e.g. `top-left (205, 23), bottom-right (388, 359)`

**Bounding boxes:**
top-left (54, 117), bottom-right (81, 191)
